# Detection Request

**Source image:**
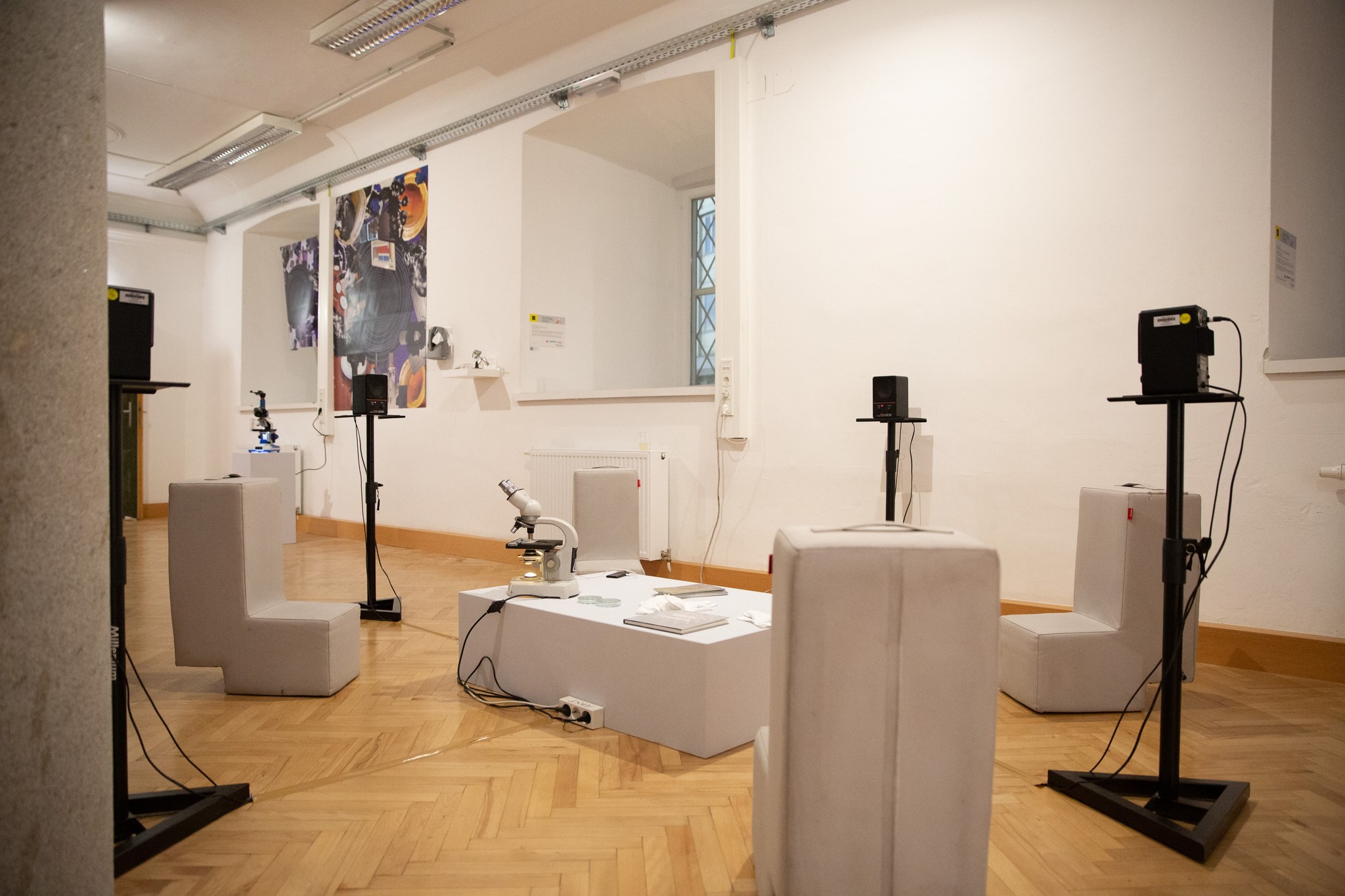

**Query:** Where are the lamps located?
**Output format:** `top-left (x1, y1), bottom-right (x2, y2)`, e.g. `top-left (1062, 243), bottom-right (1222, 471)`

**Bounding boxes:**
top-left (573, 70), bottom-right (620, 97)
top-left (144, 113), bottom-right (302, 191)
top-left (309, 0), bottom-right (469, 61)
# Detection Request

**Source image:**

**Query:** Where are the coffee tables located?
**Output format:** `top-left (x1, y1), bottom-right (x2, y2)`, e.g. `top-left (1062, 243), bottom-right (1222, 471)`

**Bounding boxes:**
top-left (458, 570), bottom-right (773, 759)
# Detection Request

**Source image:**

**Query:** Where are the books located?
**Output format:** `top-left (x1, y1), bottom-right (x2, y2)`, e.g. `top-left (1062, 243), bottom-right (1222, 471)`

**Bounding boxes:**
top-left (623, 609), bottom-right (730, 635)
top-left (658, 584), bottom-right (728, 599)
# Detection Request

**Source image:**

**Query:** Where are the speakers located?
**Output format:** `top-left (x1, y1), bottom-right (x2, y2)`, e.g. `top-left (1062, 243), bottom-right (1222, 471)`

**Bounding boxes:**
top-left (1138, 305), bottom-right (1210, 395)
top-left (872, 376), bottom-right (909, 418)
top-left (108, 285), bottom-right (154, 379)
top-left (352, 374), bottom-right (388, 415)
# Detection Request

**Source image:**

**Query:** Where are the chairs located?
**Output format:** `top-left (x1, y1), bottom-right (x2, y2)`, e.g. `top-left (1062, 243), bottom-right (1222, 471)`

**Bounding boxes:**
top-left (753, 521), bottom-right (999, 895)
top-left (572, 466), bottom-right (645, 575)
top-left (168, 473), bottom-right (360, 696)
top-left (999, 483), bottom-right (1201, 712)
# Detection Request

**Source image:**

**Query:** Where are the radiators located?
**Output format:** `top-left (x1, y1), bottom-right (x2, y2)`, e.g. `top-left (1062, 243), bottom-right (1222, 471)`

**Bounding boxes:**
top-left (530, 449), bottom-right (669, 561)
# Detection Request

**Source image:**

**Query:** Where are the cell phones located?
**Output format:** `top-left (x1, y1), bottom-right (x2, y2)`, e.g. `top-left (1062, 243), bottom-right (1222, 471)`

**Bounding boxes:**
top-left (606, 572), bottom-right (630, 578)
